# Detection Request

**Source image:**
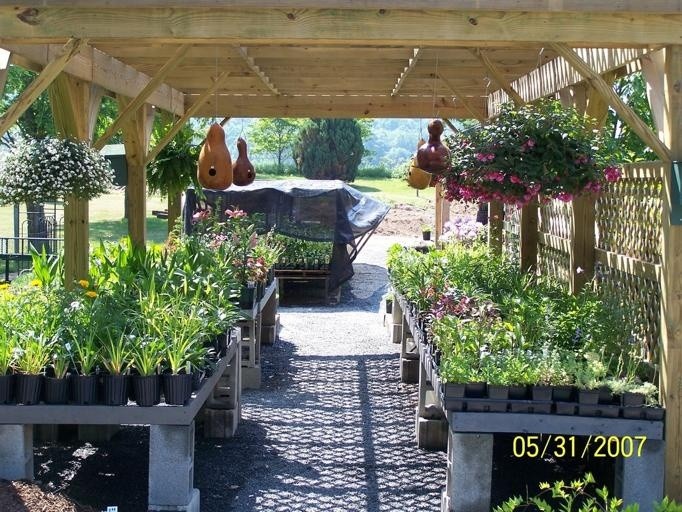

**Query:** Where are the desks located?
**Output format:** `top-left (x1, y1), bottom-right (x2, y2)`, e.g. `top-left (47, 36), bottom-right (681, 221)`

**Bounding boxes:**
top-left (236, 278), bottom-right (280, 389)
top-left (0, 327), bottom-right (243, 512)
top-left (416, 361), bottom-right (666, 512)
top-left (275, 270), bottom-right (340, 306)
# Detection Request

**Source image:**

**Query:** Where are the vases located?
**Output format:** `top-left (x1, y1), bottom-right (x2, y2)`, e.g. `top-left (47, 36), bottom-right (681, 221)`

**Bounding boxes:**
top-left (235, 268), bottom-right (277, 307)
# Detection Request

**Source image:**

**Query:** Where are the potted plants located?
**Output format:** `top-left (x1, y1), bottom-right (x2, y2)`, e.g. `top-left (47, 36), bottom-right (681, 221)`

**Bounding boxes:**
top-left (386, 242), bottom-right (664, 420)
top-left (0, 232), bottom-right (243, 405)
top-left (423, 225), bottom-right (431, 240)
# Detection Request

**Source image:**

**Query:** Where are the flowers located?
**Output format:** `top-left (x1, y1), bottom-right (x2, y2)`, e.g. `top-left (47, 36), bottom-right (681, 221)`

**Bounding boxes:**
top-left (436, 104), bottom-right (622, 209)
top-left (174, 204), bottom-right (289, 288)
top-left (0, 134), bottom-right (115, 207)
